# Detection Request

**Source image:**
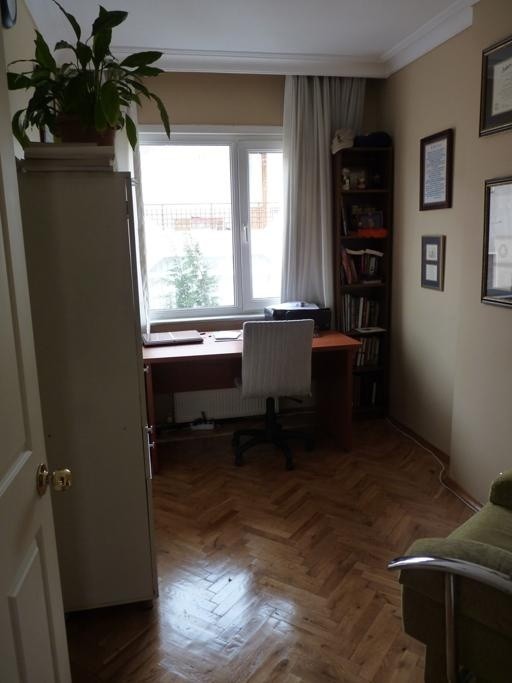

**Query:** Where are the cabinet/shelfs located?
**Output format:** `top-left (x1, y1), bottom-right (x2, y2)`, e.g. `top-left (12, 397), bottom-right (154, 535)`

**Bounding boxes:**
top-left (13, 166), bottom-right (159, 613)
top-left (330, 143), bottom-right (394, 421)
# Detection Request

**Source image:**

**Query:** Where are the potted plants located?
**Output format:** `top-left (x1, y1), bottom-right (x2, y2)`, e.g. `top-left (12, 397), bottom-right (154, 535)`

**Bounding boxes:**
top-left (8, 1), bottom-right (172, 149)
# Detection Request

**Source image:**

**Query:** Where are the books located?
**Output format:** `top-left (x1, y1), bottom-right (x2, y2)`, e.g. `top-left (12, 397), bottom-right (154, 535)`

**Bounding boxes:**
top-left (340, 248), bottom-right (387, 407)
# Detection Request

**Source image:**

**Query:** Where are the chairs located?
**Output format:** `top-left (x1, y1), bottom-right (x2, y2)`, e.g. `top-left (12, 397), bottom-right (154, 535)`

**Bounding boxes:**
top-left (385, 469), bottom-right (511, 682)
top-left (230, 318), bottom-right (315, 470)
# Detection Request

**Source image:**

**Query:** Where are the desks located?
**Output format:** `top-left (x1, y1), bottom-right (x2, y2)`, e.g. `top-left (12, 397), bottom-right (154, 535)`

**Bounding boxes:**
top-left (143, 328), bottom-right (362, 473)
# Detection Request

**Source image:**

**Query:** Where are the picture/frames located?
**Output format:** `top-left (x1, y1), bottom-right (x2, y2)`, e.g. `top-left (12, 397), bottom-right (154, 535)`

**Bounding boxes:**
top-left (418, 32), bottom-right (511, 310)
